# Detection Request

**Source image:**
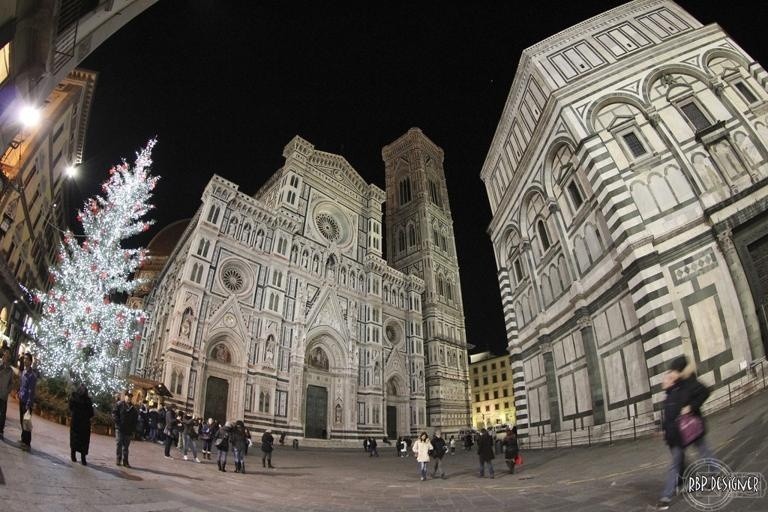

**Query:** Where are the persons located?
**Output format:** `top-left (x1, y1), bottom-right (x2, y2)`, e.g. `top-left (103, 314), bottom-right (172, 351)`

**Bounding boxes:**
top-left (428, 430), bottom-right (446, 479)
top-left (463, 431), bottom-right (474, 450)
top-left (656, 356), bottom-right (710, 511)
top-left (230, 421), bottom-right (251, 473)
top-left (363, 437), bottom-right (379, 457)
top-left (412, 432), bottom-right (434, 480)
top-left (112, 390), bottom-right (137, 467)
top-left (136, 400), bottom-right (233, 472)
top-left (449, 435), bottom-right (456, 456)
top-left (279, 430), bottom-right (286, 445)
top-left (68, 386), bottom-right (94, 465)
top-left (383, 437), bottom-right (390, 443)
top-left (477, 429), bottom-right (495, 479)
top-left (0, 340), bottom-right (37, 452)
top-left (262, 429), bottom-right (274, 468)
top-left (396, 436), bottom-right (412, 458)
top-left (503, 426), bottom-right (519, 474)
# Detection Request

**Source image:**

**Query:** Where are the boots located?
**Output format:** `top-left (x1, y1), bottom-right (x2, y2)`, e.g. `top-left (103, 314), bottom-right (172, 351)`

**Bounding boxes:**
top-left (267, 458), bottom-right (274, 468)
top-left (234, 462), bottom-right (240, 473)
top-left (201, 449), bottom-right (212, 460)
top-left (262, 458), bottom-right (266, 468)
top-left (240, 461), bottom-right (246, 473)
top-left (217, 459), bottom-right (221, 471)
top-left (221, 461), bottom-right (227, 472)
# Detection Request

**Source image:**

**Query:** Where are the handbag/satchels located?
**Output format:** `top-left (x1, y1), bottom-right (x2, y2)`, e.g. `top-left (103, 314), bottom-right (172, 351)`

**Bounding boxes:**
top-left (200, 430), bottom-right (212, 441)
top-left (214, 438), bottom-right (224, 449)
top-left (677, 412), bottom-right (704, 445)
top-left (162, 423), bottom-right (176, 440)
top-left (513, 453), bottom-right (523, 465)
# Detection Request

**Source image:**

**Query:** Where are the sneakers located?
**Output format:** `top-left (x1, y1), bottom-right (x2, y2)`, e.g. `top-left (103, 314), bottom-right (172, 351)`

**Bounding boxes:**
top-left (164, 448), bottom-right (201, 463)
top-left (115, 458), bottom-right (132, 469)
top-left (17, 438), bottom-right (32, 450)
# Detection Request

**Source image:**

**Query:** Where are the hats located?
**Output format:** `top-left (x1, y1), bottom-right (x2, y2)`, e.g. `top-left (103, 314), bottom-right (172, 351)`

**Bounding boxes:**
top-left (669, 355), bottom-right (688, 374)
top-left (124, 389), bottom-right (134, 398)
